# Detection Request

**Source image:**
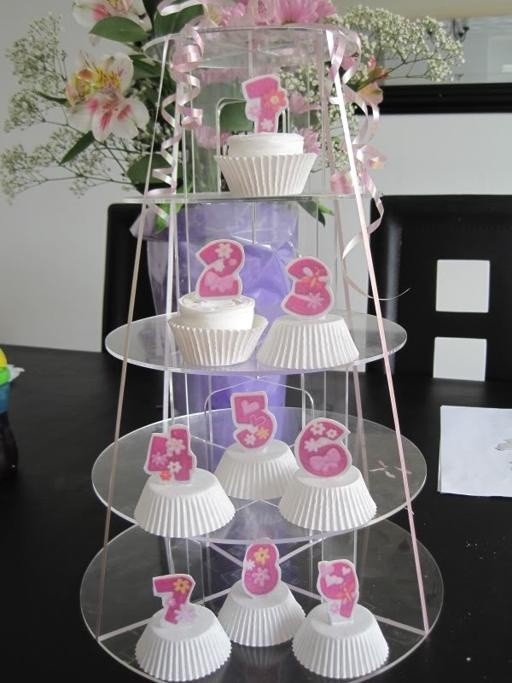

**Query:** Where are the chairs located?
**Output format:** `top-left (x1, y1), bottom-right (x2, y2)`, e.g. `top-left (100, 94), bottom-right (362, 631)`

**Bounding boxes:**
top-left (367, 188), bottom-right (512, 385)
top-left (98, 197), bottom-right (210, 363)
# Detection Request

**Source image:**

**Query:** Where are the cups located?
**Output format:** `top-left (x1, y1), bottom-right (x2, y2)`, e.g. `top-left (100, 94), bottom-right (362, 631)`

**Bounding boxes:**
top-left (262, 314), bottom-right (359, 368)
top-left (279, 463), bottom-right (379, 534)
top-left (167, 315), bottom-right (268, 366)
top-left (214, 438), bottom-right (299, 502)
top-left (219, 578), bottom-right (302, 648)
top-left (135, 605), bottom-right (231, 682)
top-left (291, 601), bottom-right (390, 678)
top-left (131, 470), bottom-right (233, 540)
top-left (212, 151), bottom-right (318, 197)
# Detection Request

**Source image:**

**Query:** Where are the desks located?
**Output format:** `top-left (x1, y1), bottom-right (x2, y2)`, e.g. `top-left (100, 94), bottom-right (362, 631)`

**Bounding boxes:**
top-left (0, 343), bottom-right (512, 682)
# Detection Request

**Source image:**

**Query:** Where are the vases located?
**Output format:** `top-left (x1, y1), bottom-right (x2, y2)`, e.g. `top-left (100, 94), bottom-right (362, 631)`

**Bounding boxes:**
top-left (129, 196), bottom-right (304, 602)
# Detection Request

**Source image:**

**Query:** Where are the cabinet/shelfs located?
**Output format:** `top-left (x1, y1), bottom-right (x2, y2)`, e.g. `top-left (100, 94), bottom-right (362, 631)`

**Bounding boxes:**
top-left (78, 18), bottom-right (445, 682)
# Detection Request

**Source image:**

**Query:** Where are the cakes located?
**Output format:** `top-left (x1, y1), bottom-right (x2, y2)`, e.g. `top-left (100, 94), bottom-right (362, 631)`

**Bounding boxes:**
top-left (224, 131), bottom-right (303, 157)
top-left (175, 289), bottom-right (254, 331)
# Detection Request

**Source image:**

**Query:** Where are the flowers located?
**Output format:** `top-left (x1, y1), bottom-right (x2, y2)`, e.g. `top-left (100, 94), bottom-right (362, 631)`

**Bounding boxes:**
top-left (0, 0), bottom-right (465, 224)
